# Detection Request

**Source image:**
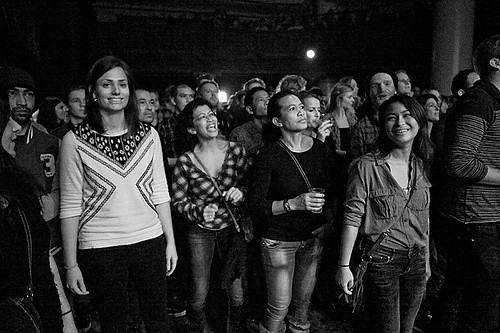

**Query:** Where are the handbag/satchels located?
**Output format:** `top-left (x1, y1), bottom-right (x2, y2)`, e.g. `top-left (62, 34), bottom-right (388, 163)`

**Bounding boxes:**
top-left (331, 261), bottom-right (368, 317)
top-left (0, 297), bottom-right (45, 333)
top-left (240, 217), bottom-right (254, 243)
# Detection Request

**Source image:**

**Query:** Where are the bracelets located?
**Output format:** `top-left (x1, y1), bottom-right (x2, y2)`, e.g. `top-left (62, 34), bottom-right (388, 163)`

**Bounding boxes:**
top-left (336, 262), bottom-right (351, 268)
top-left (63, 264), bottom-right (78, 270)
top-left (59, 309), bottom-right (73, 317)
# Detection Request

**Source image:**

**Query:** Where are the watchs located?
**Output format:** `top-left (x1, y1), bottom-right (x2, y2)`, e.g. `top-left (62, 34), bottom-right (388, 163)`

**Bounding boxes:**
top-left (283, 197), bottom-right (293, 214)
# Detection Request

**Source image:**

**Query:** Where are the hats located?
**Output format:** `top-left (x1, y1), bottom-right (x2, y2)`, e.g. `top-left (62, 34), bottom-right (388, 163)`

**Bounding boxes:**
top-left (7, 66), bottom-right (36, 90)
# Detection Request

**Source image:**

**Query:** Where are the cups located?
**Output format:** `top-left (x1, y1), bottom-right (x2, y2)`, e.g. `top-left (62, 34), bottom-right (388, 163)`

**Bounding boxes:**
top-left (309, 188), bottom-right (325, 213)
top-left (317, 117), bottom-right (332, 133)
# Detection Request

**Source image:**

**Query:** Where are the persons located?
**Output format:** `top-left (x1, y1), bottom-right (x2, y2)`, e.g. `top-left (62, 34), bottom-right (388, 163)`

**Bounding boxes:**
top-left (36, 98), bottom-right (68, 134)
top-left (245, 89), bottom-right (329, 333)
top-left (441, 34), bottom-right (500, 333)
top-left (156, 84), bottom-right (195, 165)
top-left (334, 93), bottom-right (432, 333)
top-left (170, 98), bottom-right (254, 333)
top-left (53, 83), bottom-right (89, 139)
top-left (0, 67), bottom-right (92, 333)
top-left (346, 71), bottom-right (399, 170)
top-left (133, 88), bottom-right (172, 130)
top-left (224, 78), bottom-right (271, 157)
top-left (269, 75), bottom-right (361, 196)
top-left (394, 68), bottom-right (484, 211)
top-left (59, 55), bottom-right (178, 333)
top-left (196, 74), bottom-right (236, 142)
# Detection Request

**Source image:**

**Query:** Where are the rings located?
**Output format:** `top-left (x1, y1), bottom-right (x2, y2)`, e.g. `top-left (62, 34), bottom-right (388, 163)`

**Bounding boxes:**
top-left (209, 215), bottom-right (212, 219)
